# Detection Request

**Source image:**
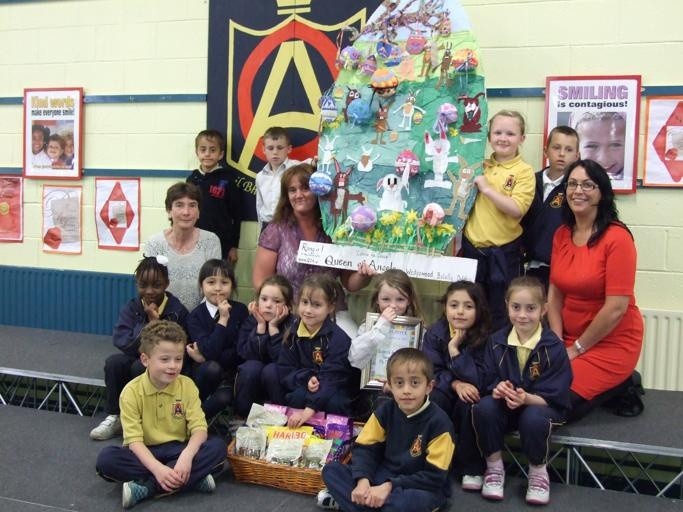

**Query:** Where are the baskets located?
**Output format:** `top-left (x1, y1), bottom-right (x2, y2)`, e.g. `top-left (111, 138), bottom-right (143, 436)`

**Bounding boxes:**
top-left (227, 421), bottom-right (365, 494)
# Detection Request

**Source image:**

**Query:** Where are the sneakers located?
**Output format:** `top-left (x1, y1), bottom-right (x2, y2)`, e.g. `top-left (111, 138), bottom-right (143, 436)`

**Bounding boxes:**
top-left (525, 471), bottom-right (551, 505)
top-left (462, 476), bottom-right (481, 491)
top-left (315, 488), bottom-right (343, 512)
top-left (90, 414), bottom-right (122, 440)
top-left (122, 480), bottom-right (149, 509)
top-left (191, 473), bottom-right (216, 494)
top-left (481, 468), bottom-right (506, 500)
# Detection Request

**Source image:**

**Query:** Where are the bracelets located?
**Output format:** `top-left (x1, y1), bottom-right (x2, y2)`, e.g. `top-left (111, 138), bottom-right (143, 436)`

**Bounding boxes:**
top-left (574, 338), bottom-right (585, 356)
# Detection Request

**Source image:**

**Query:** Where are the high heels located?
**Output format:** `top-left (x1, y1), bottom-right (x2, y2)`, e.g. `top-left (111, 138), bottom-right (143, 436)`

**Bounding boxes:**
top-left (618, 370), bottom-right (646, 416)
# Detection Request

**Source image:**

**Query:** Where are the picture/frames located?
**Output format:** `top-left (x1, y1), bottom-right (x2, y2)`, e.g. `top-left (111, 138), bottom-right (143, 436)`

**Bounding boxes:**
top-left (360, 310), bottom-right (424, 393)
top-left (538, 73), bottom-right (640, 196)
top-left (21, 87), bottom-right (83, 182)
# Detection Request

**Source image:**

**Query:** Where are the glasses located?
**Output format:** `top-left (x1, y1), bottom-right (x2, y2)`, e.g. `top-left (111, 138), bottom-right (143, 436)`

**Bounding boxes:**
top-left (567, 181), bottom-right (598, 192)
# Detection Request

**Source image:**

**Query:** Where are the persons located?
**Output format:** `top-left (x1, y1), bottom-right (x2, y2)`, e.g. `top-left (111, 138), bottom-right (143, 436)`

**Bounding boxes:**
top-left (543, 157), bottom-right (644, 426)
top-left (420, 279), bottom-right (493, 492)
top-left (88, 254), bottom-right (192, 442)
top-left (141, 180), bottom-right (223, 313)
top-left (347, 267), bottom-right (424, 418)
top-left (43, 133), bottom-right (66, 170)
top-left (523, 125), bottom-right (581, 298)
top-left (232, 272), bottom-right (298, 436)
top-left (314, 345), bottom-right (459, 511)
top-left (568, 112), bottom-right (627, 181)
top-left (467, 274), bottom-right (573, 506)
top-left (183, 129), bottom-right (242, 275)
top-left (459, 109), bottom-right (538, 333)
top-left (253, 125), bottom-right (320, 232)
top-left (176, 259), bottom-right (250, 422)
top-left (29, 124), bottom-right (52, 171)
top-left (93, 318), bottom-right (232, 509)
top-left (274, 270), bottom-right (358, 421)
top-left (248, 163), bottom-right (377, 342)
top-left (59, 130), bottom-right (73, 170)
